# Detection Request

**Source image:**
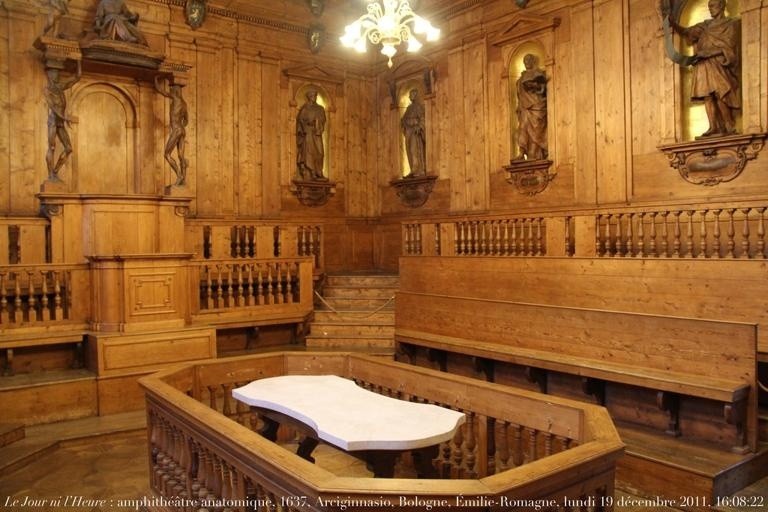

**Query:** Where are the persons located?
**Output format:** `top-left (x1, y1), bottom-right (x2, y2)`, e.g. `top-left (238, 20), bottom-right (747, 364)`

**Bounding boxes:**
top-left (153, 75), bottom-right (188, 185)
top-left (95, 0), bottom-right (149, 47)
top-left (296, 89), bottom-right (326, 181)
top-left (44, 57), bottom-right (82, 182)
top-left (44, 0), bottom-right (70, 35)
top-left (401, 90), bottom-right (426, 177)
top-left (671, 0), bottom-right (742, 137)
top-left (511, 55), bottom-right (549, 162)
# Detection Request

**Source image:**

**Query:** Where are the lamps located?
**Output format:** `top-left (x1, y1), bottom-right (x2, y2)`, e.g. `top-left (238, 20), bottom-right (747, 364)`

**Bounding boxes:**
top-left (339, 0), bottom-right (441, 70)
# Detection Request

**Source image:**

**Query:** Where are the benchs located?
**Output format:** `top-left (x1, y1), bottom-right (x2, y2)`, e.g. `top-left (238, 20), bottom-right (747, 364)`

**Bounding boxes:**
top-left (393, 328), bottom-right (751, 455)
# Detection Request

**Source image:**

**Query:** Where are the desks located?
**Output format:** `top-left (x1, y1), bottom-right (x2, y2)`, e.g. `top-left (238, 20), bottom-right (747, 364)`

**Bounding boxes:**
top-left (231, 375), bottom-right (467, 479)
